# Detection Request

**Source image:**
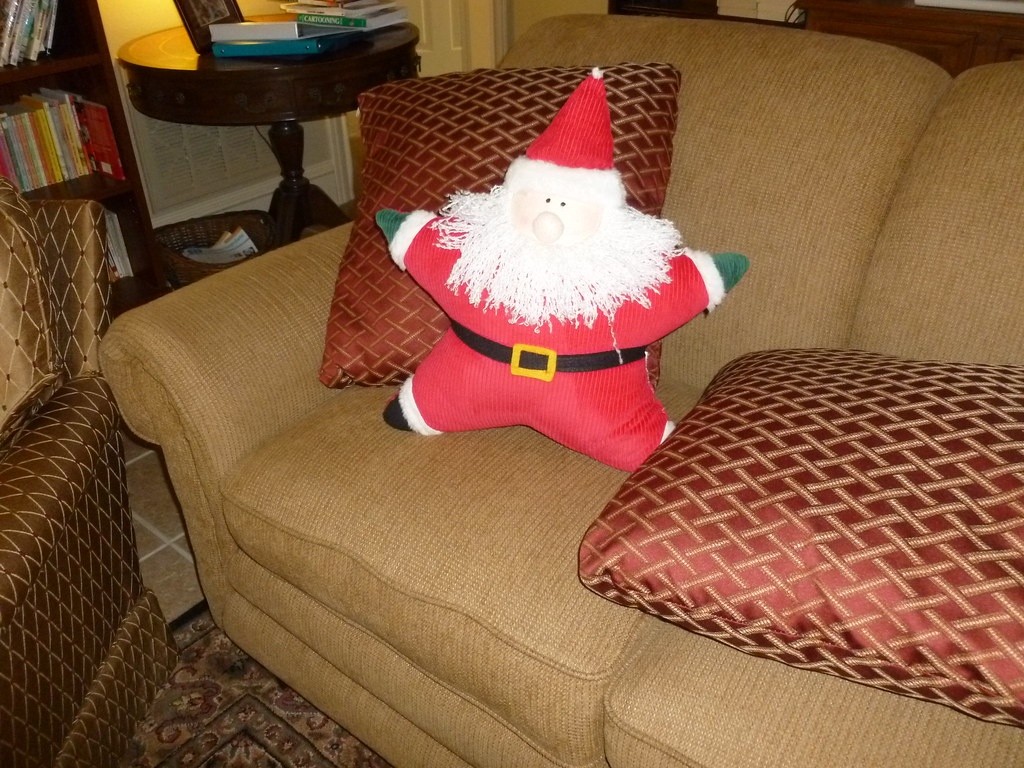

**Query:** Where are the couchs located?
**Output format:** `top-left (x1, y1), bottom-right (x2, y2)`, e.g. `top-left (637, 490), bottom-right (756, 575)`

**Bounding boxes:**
top-left (97, 15), bottom-right (1024, 767)
top-left (0, 183), bottom-right (176, 768)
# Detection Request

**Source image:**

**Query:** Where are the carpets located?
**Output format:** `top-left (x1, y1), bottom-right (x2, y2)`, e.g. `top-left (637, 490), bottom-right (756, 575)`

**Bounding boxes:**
top-left (155, 598), bottom-right (399, 767)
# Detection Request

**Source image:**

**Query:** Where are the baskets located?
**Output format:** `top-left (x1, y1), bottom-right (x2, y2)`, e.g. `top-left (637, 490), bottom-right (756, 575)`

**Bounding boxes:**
top-left (151, 209), bottom-right (275, 287)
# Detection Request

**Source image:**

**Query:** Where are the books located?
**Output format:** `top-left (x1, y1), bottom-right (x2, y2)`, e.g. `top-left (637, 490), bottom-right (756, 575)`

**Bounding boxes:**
top-left (105, 212), bottom-right (134, 279)
top-left (0, 0), bottom-right (58, 67)
top-left (279, 0), bottom-right (406, 29)
top-left (0, 88), bottom-right (126, 194)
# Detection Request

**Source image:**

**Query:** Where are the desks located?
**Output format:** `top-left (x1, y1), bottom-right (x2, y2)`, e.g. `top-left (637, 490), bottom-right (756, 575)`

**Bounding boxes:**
top-left (117, 23), bottom-right (425, 246)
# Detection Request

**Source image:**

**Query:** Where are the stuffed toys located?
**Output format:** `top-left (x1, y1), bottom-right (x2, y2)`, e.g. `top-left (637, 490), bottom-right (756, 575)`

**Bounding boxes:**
top-left (376, 66), bottom-right (750, 473)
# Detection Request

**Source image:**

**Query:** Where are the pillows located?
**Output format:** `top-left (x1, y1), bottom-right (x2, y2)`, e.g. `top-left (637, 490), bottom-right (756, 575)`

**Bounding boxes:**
top-left (0, 185), bottom-right (66, 434)
top-left (578, 346), bottom-right (1022, 724)
top-left (321, 57), bottom-right (677, 384)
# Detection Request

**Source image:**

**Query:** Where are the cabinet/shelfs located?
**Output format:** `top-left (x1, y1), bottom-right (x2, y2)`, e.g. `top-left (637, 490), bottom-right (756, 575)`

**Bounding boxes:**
top-left (0, 0), bottom-right (171, 304)
